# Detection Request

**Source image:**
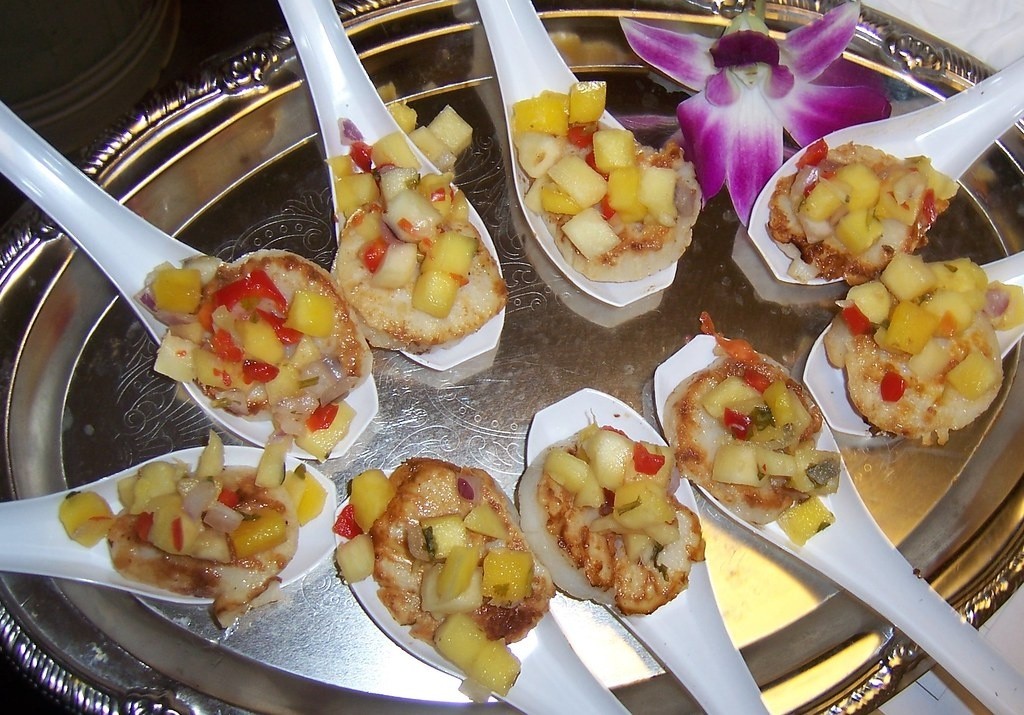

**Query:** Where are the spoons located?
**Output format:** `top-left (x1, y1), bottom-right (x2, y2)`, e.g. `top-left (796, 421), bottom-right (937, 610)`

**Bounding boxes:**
top-left (747, 57), bottom-right (1024, 286)
top-left (653, 334), bottom-right (1024, 715)
top-left (0, 100), bottom-right (379, 460)
top-left (476, 0), bottom-right (678, 308)
top-left (334, 469), bottom-right (634, 715)
top-left (803, 250), bottom-right (1024, 436)
top-left (276, 0), bottom-right (506, 372)
top-left (526, 387), bottom-right (771, 715)
top-left (0, 445), bottom-right (337, 604)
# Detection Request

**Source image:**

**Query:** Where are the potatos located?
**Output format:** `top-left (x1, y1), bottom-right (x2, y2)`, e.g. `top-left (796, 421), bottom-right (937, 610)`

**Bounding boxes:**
top-left (58, 82), bottom-right (1024, 697)
